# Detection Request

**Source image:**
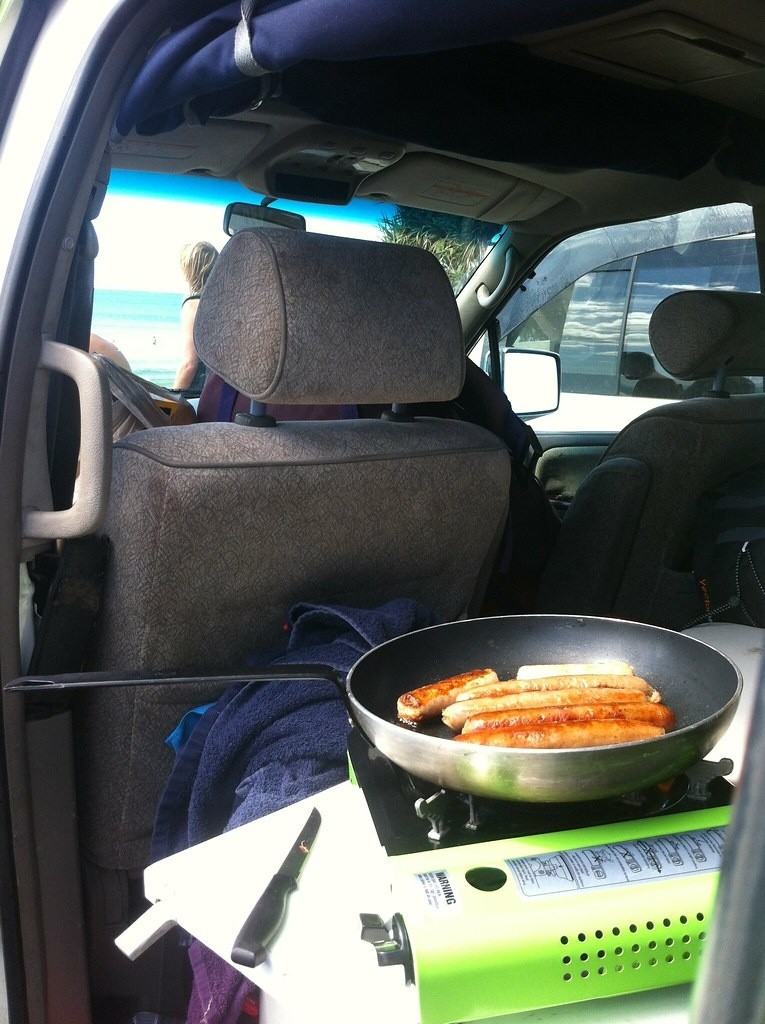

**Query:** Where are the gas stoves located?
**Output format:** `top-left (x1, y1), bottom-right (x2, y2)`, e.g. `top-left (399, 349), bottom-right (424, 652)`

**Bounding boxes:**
top-left (345, 732), bottom-right (740, 1024)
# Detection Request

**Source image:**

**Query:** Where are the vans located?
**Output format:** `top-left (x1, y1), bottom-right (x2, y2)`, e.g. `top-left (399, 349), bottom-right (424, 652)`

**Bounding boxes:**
top-left (9, 0), bottom-right (765, 1024)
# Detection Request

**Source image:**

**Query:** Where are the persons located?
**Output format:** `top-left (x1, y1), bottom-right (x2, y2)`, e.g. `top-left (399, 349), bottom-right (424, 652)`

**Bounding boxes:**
top-left (620, 351), bottom-right (677, 399)
top-left (90, 334), bottom-right (133, 372)
top-left (173, 241), bottom-right (218, 400)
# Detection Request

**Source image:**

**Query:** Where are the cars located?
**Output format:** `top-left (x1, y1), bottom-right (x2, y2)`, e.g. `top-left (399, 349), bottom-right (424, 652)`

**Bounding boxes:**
top-left (465, 199), bottom-right (762, 445)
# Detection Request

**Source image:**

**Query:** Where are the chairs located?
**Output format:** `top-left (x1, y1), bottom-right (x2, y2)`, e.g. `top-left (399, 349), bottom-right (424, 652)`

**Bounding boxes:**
top-left (536, 287), bottom-right (759, 624)
top-left (74, 225), bottom-right (517, 868)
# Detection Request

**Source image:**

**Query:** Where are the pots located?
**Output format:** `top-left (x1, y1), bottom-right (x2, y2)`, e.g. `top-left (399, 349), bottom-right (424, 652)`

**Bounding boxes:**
top-left (0, 615), bottom-right (742, 804)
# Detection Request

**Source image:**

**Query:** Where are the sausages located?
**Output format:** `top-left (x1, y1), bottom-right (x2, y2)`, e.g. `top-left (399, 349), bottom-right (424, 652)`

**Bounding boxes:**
top-left (396, 659), bottom-right (675, 750)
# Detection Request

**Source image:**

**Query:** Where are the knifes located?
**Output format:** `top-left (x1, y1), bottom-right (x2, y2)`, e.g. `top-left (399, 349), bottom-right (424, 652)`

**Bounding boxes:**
top-left (232, 807), bottom-right (322, 968)
